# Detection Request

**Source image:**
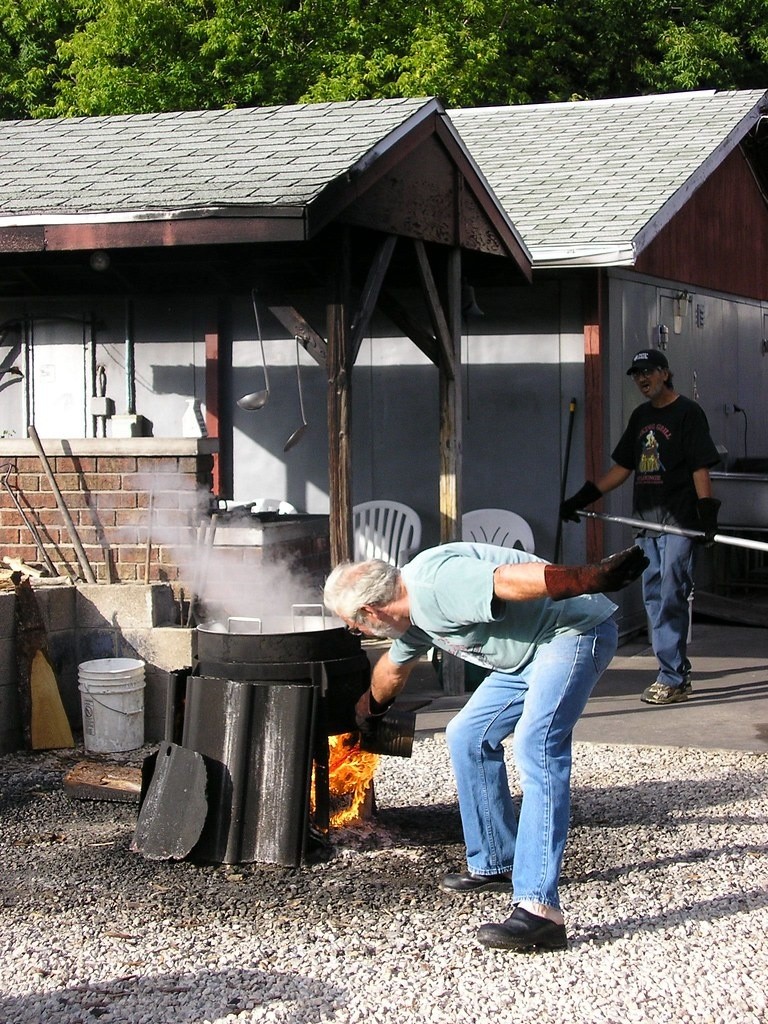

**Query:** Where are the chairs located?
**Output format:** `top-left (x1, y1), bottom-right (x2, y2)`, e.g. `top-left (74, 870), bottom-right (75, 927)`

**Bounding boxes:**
top-left (352, 501), bottom-right (422, 570)
top-left (440, 508), bottom-right (534, 554)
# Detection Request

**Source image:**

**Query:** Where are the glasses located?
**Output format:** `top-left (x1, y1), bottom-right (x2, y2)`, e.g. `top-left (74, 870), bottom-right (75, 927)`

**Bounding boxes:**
top-left (630, 367), bottom-right (664, 380)
top-left (348, 610), bottom-right (363, 636)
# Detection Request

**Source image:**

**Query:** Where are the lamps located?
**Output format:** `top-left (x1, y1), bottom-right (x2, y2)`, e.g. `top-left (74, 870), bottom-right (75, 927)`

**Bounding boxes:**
top-left (678, 288), bottom-right (689, 316)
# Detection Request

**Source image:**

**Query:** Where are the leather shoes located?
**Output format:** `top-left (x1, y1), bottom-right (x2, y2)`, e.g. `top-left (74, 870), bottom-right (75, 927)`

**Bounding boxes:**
top-left (477, 907), bottom-right (567, 950)
top-left (442, 871), bottom-right (513, 893)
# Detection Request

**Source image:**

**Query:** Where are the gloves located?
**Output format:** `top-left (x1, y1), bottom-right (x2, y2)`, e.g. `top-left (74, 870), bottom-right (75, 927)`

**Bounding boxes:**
top-left (544, 545), bottom-right (651, 602)
top-left (560, 480), bottom-right (603, 523)
top-left (354, 687), bottom-right (397, 740)
top-left (695, 498), bottom-right (722, 548)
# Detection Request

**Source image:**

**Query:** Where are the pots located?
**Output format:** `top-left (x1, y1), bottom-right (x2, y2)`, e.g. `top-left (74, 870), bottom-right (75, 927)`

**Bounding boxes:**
top-left (196, 599), bottom-right (366, 734)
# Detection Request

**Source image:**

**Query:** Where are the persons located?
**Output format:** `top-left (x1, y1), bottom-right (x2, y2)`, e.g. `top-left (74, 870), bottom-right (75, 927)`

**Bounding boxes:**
top-left (323, 541), bottom-right (652, 951)
top-left (559, 348), bottom-right (722, 704)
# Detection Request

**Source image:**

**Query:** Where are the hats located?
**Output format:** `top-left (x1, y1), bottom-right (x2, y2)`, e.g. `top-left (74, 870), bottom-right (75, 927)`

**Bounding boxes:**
top-left (627, 350), bottom-right (668, 375)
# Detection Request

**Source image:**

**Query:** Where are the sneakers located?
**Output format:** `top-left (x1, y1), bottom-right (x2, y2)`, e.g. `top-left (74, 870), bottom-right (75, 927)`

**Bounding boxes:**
top-left (683, 674), bottom-right (693, 695)
top-left (640, 681), bottom-right (688, 704)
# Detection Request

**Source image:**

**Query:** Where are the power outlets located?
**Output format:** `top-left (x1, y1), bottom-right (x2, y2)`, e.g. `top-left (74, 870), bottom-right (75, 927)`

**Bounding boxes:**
top-left (726, 404), bottom-right (737, 414)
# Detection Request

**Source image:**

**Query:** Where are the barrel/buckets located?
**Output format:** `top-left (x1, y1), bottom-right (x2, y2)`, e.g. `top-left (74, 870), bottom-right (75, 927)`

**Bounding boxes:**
top-left (76, 657), bottom-right (146, 754)
